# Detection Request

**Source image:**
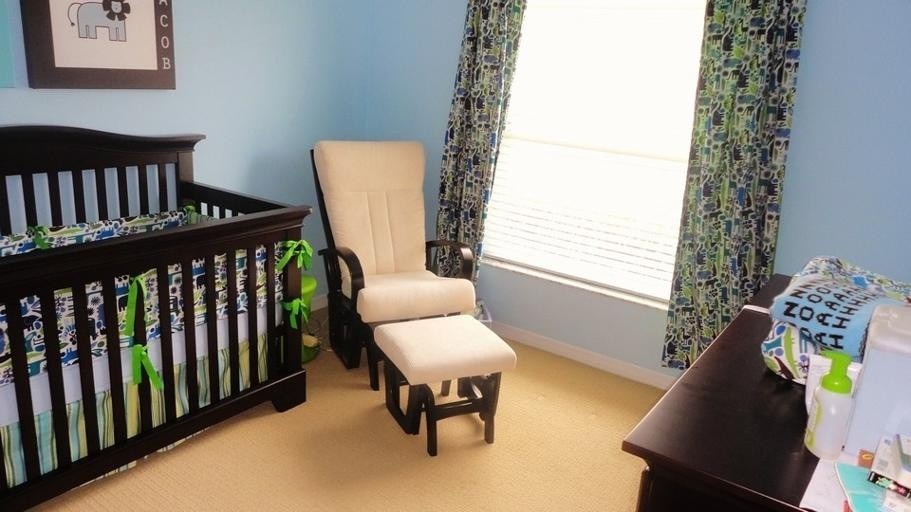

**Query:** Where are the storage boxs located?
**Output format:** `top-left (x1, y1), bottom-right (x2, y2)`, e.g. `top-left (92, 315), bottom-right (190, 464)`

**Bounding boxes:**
top-left (846, 303), bottom-right (911, 459)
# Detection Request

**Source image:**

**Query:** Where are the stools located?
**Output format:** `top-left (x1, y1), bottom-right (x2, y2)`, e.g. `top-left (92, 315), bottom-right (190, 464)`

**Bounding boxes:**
top-left (374, 317), bottom-right (520, 455)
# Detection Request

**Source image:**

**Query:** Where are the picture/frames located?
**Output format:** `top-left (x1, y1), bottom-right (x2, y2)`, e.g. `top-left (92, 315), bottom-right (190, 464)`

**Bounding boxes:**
top-left (18, 0), bottom-right (177, 89)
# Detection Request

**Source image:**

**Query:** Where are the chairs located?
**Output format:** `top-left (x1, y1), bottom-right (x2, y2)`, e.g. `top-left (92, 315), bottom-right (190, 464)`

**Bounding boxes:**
top-left (307, 138), bottom-right (477, 389)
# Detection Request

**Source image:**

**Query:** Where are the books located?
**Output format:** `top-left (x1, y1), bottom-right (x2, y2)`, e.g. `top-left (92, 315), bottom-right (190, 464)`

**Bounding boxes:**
top-left (834, 434), bottom-right (911, 512)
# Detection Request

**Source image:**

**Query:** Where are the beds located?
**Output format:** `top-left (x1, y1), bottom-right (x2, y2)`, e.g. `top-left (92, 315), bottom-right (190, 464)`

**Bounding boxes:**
top-left (1, 126), bottom-right (309, 512)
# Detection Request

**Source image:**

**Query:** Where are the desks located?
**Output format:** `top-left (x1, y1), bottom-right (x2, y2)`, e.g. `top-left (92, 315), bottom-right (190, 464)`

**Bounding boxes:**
top-left (620, 272), bottom-right (853, 511)
